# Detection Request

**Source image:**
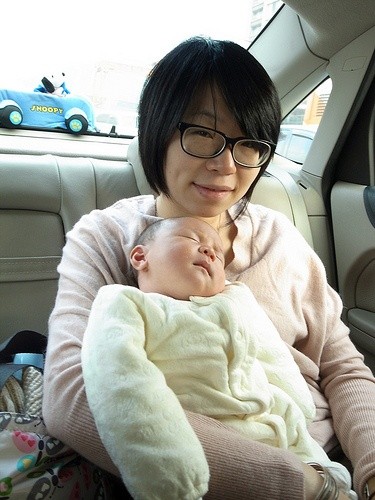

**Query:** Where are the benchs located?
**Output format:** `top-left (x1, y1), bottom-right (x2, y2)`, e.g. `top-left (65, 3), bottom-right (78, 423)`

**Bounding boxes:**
top-left (1, 127), bottom-right (341, 363)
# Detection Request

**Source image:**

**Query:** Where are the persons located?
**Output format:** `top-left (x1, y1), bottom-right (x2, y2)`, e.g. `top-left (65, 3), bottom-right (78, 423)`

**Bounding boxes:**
top-left (41, 38), bottom-right (374, 500)
top-left (77, 216), bottom-right (357, 500)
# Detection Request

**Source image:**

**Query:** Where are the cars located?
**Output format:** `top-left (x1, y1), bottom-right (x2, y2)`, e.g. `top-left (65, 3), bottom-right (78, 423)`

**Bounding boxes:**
top-left (0, 76), bottom-right (98, 136)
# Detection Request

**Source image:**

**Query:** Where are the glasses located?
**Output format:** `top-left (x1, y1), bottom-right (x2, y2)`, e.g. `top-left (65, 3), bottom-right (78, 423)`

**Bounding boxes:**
top-left (175, 122), bottom-right (274, 168)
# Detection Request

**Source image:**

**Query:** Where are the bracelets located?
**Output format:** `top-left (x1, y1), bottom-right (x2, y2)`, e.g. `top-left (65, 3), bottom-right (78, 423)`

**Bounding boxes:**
top-left (307, 461), bottom-right (340, 500)
top-left (362, 479), bottom-right (375, 500)
